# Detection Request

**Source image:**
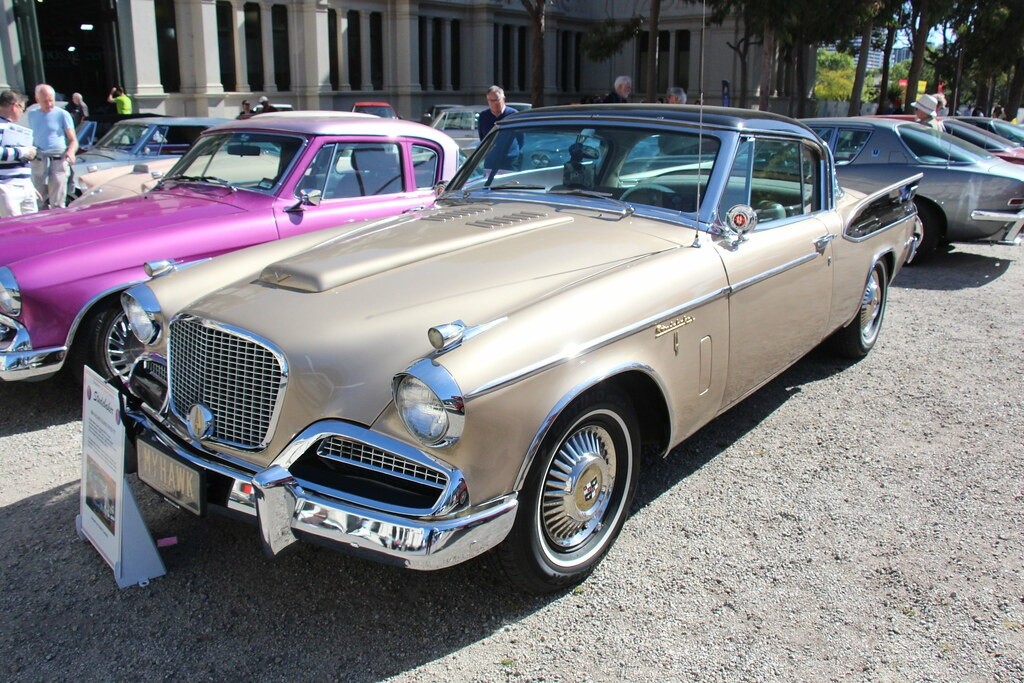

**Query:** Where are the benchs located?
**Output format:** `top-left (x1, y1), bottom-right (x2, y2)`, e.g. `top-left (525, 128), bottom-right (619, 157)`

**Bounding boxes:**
top-left (377, 153), bottom-right (436, 193)
top-left (636, 180), bottom-right (810, 210)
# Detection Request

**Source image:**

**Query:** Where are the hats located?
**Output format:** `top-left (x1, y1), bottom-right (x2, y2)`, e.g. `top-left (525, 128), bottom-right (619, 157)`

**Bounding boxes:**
top-left (911, 96), bottom-right (938, 116)
top-left (259, 96), bottom-right (268, 103)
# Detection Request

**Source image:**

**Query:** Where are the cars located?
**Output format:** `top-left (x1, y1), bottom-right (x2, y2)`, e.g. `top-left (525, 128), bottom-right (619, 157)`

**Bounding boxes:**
top-left (70, 116), bottom-right (239, 202)
top-left (427, 108), bottom-right (482, 161)
top-left (251, 104), bottom-right (295, 113)
top-left (119, 105), bottom-right (926, 587)
top-left (506, 103), bottom-right (533, 112)
top-left (861, 113), bottom-right (1024, 165)
top-left (423, 104), bottom-right (464, 121)
top-left (619, 117), bottom-right (1024, 267)
top-left (957, 116), bottom-right (1024, 146)
top-left (355, 101), bottom-right (399, 121)
top-left (0, 119), bottom-right (520, 394)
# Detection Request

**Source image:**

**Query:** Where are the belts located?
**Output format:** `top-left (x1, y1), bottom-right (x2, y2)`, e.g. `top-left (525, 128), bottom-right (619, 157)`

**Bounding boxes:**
top-left (37, 157), bottom-right (61, 160)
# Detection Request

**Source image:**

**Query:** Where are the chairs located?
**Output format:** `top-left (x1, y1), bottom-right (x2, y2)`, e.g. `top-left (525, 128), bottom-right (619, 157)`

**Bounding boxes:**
top-left (333, 143), bottom-right (386, 199)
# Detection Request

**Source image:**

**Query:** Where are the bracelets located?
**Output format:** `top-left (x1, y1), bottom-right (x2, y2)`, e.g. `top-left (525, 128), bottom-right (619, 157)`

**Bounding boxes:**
top-left (110, 93), bottom-right (113, 95)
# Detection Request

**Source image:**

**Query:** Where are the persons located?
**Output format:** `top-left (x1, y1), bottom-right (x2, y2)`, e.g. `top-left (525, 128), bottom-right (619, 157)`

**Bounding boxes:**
top-left (107, 87), bottom-right (132, 144)
top-left (72, 93), bottom-right (91, 147)
top-left (235, 100), bottom-right (256, 119)
top-left (0, 91), bottom-right (43, 217)
top-left (657, 87), bottom-right (701, 104)
top-left (603, 75), bottom-right (633, 103)
top-left (874, 93), bottom-right (1006, 161)
top-left (478, 86), bottom-right (525, 178)
top-left (259, 95), bottom-right (277, 112)
top-left (26, 84), bottom-right (78, 212)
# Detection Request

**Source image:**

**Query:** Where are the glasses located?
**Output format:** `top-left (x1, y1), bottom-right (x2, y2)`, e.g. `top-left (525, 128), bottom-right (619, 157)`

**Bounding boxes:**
top-left (18, 105), bottom-right (27, 114)
top-left (488, 99), bottom-right (500, 103)
